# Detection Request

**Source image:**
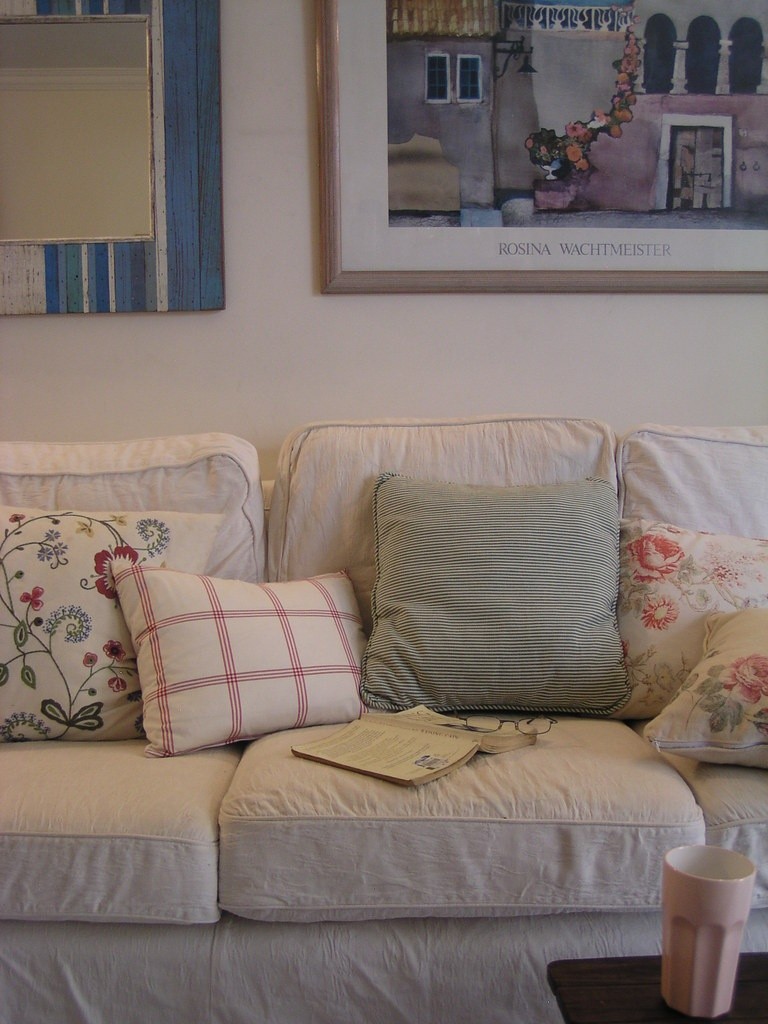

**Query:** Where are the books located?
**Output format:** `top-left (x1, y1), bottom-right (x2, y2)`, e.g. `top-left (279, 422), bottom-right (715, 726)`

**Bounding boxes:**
top-left (290, 702), bottom-right (540, 787)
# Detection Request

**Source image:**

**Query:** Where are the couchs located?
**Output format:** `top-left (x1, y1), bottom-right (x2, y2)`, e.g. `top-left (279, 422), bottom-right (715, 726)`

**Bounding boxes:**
top-left (1, 417), bottom-right (768, 1024)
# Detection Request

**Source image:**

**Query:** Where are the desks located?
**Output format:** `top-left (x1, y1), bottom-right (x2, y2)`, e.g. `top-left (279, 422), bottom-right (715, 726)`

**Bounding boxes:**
top-left (548, 951), bottom-right (768, 1023)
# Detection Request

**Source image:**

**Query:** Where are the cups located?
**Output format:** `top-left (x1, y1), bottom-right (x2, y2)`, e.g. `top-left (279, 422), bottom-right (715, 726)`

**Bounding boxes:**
top-left (659, 844), bottom-right (757, 1019)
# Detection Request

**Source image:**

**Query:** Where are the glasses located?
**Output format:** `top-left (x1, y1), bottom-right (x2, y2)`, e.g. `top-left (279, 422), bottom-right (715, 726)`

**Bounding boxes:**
top-left (458, 714), bottom-right (558, 736)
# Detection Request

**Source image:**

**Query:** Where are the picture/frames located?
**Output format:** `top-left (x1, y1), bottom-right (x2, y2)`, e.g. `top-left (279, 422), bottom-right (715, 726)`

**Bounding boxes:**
top-left (316, 0), bottom-right (768, 294)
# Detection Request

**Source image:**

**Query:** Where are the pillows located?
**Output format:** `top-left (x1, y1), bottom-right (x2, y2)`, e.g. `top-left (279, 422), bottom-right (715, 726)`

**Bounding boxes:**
top-left (360, 473), bottom-right (631, 714)
top-left (606, 518), bottom-right (768, 719)
top-left (111, 560), bottom-right (390, 757)
top-left (1, 506), bottom-right (225, 741)
top-left (643, 608), bottom-right (768, 770)
